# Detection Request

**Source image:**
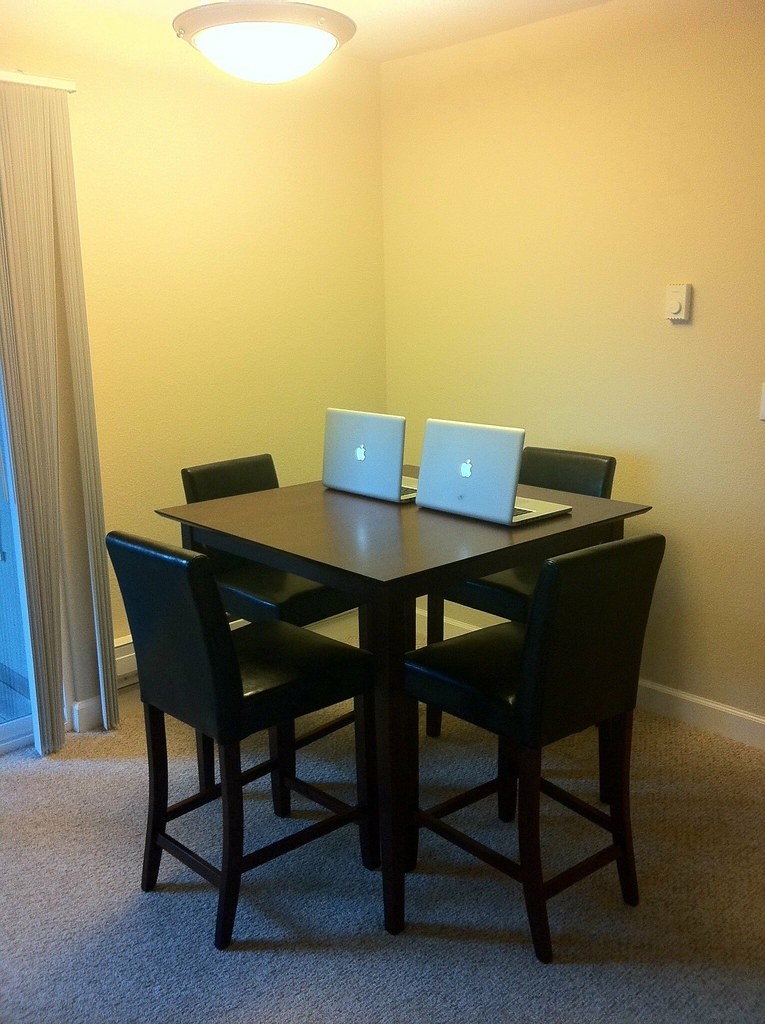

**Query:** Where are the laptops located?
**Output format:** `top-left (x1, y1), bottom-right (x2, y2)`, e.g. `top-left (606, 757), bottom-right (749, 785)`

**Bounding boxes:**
top-left (321, 407), bottom-right (420, 502)
top-left (415, 419), bottom-right (573, 527)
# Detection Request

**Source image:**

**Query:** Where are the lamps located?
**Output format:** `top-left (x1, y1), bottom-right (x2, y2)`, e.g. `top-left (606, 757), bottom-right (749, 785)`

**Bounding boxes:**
top-left (172, 0), bottom-right (356, 86)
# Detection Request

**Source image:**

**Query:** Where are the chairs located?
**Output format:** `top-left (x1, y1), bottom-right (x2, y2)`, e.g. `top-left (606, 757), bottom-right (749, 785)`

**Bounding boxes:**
top-left (182, 452), bottom-right (357, 755)
top-left (424, 448), bottom-right (616, 765)
top-left (106, 530), bottom-right (384, 952)
top-left (401, 533), bottom-right (667, 966)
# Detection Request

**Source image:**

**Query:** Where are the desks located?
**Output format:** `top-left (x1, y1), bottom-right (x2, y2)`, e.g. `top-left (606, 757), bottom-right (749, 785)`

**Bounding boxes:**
top-left (155, 462), bottom-right (653, 934)
